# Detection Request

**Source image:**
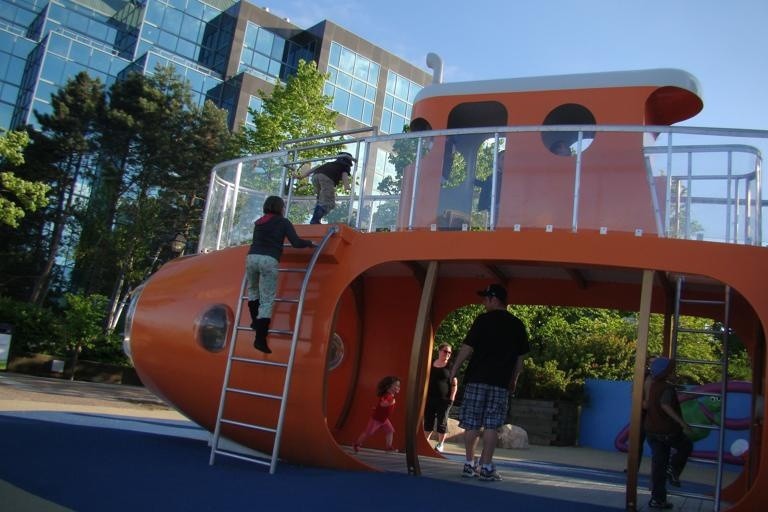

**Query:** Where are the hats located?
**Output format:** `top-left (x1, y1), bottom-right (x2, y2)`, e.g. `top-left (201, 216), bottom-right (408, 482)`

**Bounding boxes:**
top-left (338, 151), bottom-right (353, 166)
top-left (650, 357), bottom-right (672, 378)
top-left (478, 284), bottom-right (508, 306)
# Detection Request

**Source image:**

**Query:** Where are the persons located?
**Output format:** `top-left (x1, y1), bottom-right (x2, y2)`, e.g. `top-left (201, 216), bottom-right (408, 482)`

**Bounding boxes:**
top-left (353, 375), bottom-right (402, 455)
top-left (289, 150), bottom-right (357, 226)
top-left (548, 140), bottom-right (574, 157)
top-left (422, 341), bottom-right (459, 453)
top-left (473, 150), bottom-right (506, 229)
top-left (245, 194), bottom-right (320, 354)
top-left (644, 356), bottom-right (694, 510)
top-left (622, 357), bottom-right (653, 476)
top-left (447, 280), bottom-right (530, 483)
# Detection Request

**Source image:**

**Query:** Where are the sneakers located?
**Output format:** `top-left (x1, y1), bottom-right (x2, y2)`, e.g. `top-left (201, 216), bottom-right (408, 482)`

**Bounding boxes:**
top-left (385, 445), bottom-right (399, 454)
top-left (478, 462), bottom-right (503, 481)
top-left (461, 461), bottom-right (480, 478)
top-left (434, 442), bottom-right (443, 452)
top-left (351, 436), bottom-right (360, 452)
top-left (665, 464), bottom-right (681, 488)
top-left (648, 495), bottom-right (674, 510)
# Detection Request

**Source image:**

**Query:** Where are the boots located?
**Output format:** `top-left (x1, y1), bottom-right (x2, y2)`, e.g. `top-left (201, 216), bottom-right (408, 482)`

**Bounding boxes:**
top-left (248, 298), bottom-right (260, 329)
top-left (253, 317), bottom-right (272, 353)
top-left (310, 205), bottom-right (326, 225)
